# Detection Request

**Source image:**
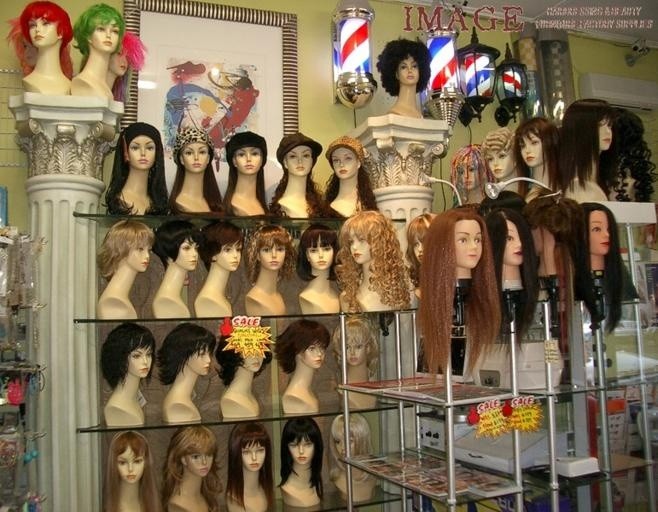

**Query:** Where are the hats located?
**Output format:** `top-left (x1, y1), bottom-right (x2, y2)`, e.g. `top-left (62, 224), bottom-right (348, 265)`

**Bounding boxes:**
top-left (326, 136), bottom-right (364, 167)
top-left (277, 134), bottom-right (322, 162)
top-left (173, 128), bottom-right (214, 166)
top-left (226, 132), bottom-right (267, 161)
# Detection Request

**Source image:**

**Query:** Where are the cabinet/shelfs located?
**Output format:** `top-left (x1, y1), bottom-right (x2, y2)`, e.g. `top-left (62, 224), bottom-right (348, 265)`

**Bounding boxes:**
top-left (539, 198), bottom-right (658, 510)
top-left (330, 300), bottom-right (531, 512)
top-left (516, 282), bottom-right (656, 511)
top-left (69, 208), bottom-right (416, 512)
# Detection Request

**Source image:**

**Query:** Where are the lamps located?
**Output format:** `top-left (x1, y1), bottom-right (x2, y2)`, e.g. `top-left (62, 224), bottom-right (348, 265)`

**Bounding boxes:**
top-left (481, 173), bottom-right (554, 201)
top-left (328, 0), bottom-right (377, 116)
top-left (454, 21), bottom-right (503, 127)
top-left (411, 169), bottom-right (467, 209)
top-left (490, 36), bottom-right (533, 128)
top-left (422, 25), bottom-right (466, 142)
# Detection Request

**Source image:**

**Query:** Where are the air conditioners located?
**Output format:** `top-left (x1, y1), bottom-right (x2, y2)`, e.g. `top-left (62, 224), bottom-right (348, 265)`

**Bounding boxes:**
top-left (577, 70), bottom-right (658, 114)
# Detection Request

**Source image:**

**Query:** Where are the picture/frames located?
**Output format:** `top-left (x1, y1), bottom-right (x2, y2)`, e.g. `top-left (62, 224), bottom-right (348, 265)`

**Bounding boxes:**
top-left (107, 0), bottom-right (298, 210)
